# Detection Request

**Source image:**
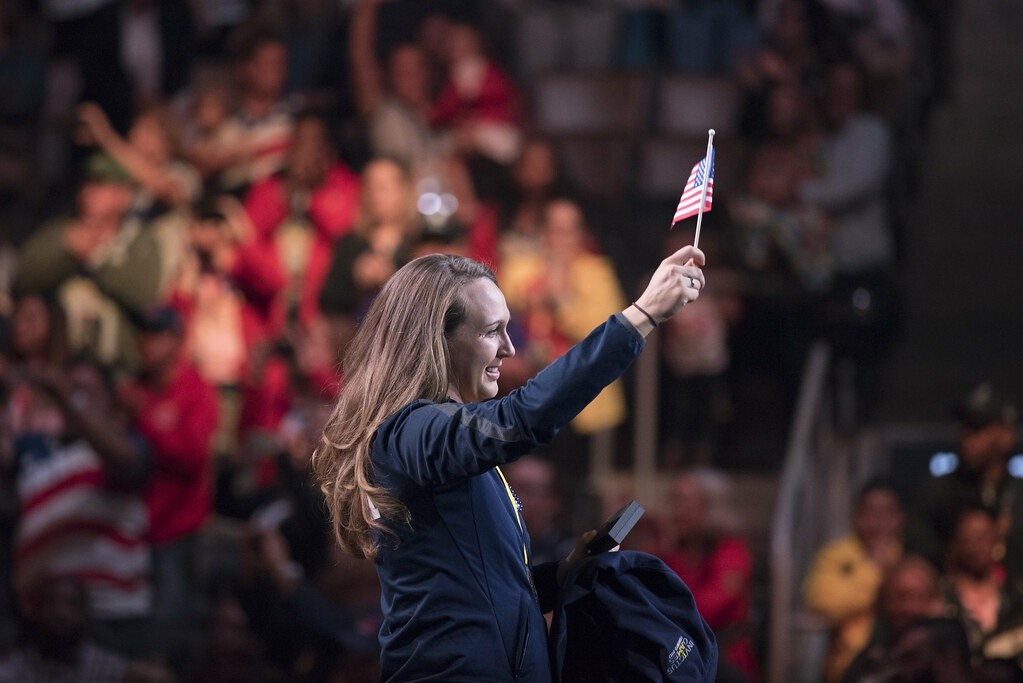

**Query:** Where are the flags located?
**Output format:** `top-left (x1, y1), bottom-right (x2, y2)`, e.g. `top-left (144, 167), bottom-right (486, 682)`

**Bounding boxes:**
top-left (669, 129), bottom-right (716, 228)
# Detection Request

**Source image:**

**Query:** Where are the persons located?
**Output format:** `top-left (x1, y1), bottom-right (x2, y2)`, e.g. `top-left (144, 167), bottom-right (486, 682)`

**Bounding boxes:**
top-left (295, 253), bottom-right (722, 683)
top-left (0, 0), bottom-right (891, 683)
top-left (802, 424), bottom-right (1023, 683)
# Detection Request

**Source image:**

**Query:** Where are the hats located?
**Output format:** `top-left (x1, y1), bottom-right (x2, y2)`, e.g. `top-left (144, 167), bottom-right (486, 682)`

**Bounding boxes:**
top-left (551, 548), bottom-right (718, 683)
